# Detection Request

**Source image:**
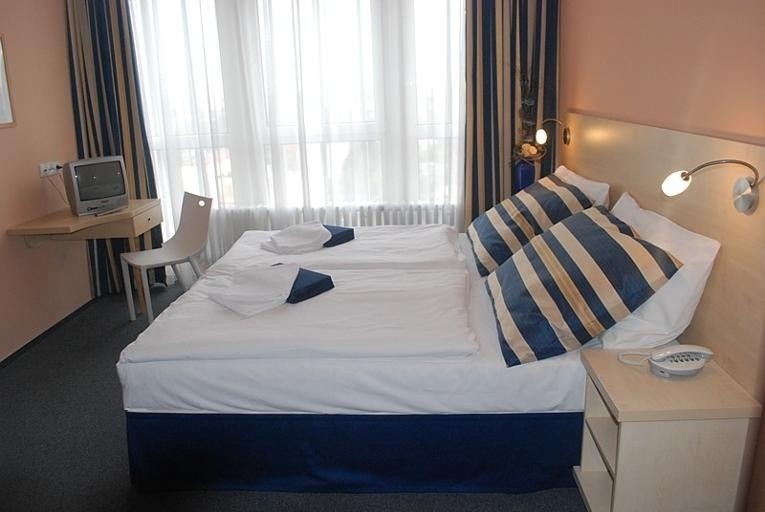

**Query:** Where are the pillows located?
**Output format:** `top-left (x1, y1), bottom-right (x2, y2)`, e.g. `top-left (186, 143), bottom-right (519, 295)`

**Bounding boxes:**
top-left (485, 204), bottom-right (683, 367)
top-left (552, 166), bottom-right (610, 211)
top-left (466, 175), bottom-right (595, 277)
top-left (596, 192), bottom-right (721, 351)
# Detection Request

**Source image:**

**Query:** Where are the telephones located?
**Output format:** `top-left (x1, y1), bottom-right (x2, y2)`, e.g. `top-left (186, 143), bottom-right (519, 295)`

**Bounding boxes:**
top-left (648, 344), bottom-right (714, 379)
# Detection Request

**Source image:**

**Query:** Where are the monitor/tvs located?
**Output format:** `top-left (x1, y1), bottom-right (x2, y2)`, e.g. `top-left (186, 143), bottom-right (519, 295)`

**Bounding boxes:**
top-left (63, 155), bottom-right (130, 216)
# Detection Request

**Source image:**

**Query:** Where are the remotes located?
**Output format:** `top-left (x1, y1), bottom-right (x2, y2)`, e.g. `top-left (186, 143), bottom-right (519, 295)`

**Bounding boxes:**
top-left (95, 206), bottom-right (124, 216)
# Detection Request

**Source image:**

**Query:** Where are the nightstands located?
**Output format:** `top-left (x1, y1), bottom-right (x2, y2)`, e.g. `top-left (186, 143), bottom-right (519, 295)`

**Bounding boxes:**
top-left (570, 342), bottom-right (765, 511)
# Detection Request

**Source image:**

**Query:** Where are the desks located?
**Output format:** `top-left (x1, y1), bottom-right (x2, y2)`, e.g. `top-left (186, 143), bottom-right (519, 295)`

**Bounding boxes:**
top-left (5, 198), bottom-right (166, 314)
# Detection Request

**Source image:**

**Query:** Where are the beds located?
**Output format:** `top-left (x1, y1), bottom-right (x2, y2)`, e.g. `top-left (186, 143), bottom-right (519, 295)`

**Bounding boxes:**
top-left (117, 111), bottom-right (764, 495)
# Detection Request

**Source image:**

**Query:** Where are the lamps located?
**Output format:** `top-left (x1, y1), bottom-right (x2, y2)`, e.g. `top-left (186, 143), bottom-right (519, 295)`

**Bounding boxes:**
top-left (535, 118), bottom-right (570, 145)
top-left (660, 151), bottom-right (761, 216)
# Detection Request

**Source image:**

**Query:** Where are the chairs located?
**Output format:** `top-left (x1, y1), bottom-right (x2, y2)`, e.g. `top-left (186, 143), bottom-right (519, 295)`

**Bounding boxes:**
top-left (119, 190), bottom-right (214, 324)
